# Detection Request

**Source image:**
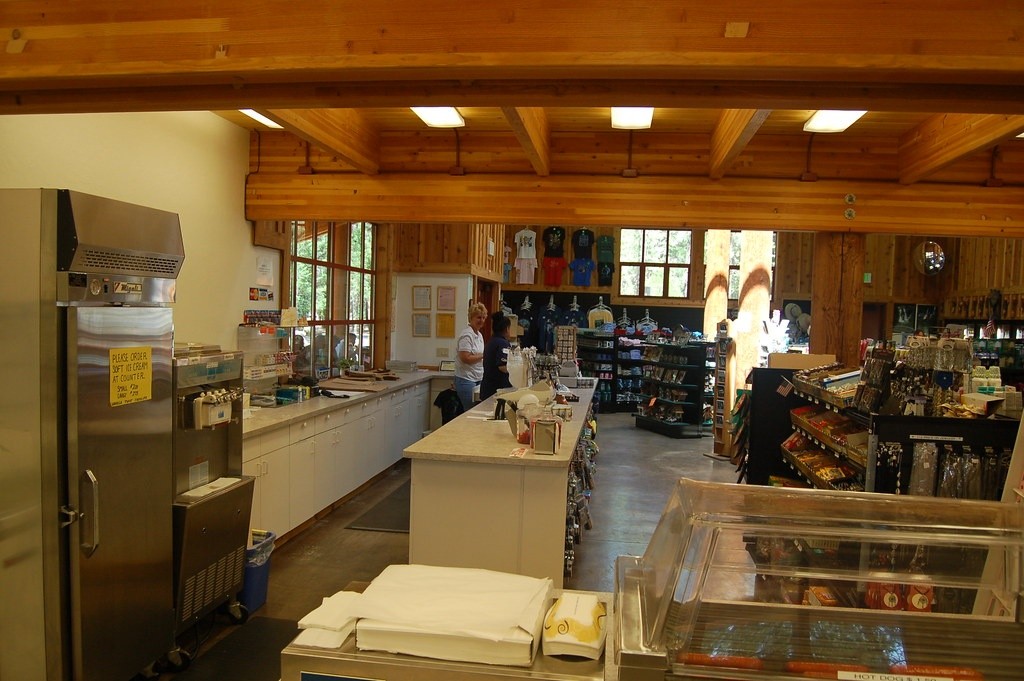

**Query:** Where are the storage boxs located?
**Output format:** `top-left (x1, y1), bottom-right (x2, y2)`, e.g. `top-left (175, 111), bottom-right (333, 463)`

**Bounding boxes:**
top-left (761, 368), bottom-right (1024, 606)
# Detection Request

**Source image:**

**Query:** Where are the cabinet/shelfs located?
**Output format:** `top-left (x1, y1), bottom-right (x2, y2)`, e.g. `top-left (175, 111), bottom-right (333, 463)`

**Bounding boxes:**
top-left (941, 290), bottom-right (1024, 393)
top-left (575, 334), bottom-right (713, 438)
top-left (237, 326), bottom-right (297, 382)
top-left (739, 367), bottom-right (1021, 615)
top-left (242, 379), bottom-right (454, 540)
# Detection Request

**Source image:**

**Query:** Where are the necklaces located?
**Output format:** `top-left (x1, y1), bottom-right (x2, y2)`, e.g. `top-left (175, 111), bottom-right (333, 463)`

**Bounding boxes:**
top-left (875, 445), bottom-right (904, 494)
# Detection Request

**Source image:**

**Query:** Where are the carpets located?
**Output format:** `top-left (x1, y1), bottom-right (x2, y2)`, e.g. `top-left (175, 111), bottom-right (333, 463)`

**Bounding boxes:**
top-left (343, 478), bottom-right (410, 533)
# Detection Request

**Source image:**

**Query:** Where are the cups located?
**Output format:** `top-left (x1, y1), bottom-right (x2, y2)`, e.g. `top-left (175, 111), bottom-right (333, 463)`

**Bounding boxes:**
top-left (242, 393), bottom-right (250, 410)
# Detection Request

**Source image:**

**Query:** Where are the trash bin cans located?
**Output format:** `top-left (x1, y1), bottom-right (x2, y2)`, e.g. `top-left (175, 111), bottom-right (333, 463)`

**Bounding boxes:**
top-left (243, 528), bottom-right (276, 613)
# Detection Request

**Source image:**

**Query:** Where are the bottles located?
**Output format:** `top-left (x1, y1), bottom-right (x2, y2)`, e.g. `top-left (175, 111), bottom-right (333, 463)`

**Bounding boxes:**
top-left (299, 313), bottom-right (307, 325)
top-left (298, 387), bottom-right (303, 404)
top-left (496, 398), bottom-right (506, 418)
top-left (973, 366), bottom-right (987, 391)
top-left (987, 364), bottom-right (1002, 387)
top-left (261, 322), bottom-right (288, 337)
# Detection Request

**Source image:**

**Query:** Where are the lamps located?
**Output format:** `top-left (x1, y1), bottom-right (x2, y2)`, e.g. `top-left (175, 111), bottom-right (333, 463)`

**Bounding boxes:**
top-left (803, 110), bottom-right (867, 133)
top-left (611, 107), bottom-right (655, 129)
top-left (410, 106), bottom-right (465, 128)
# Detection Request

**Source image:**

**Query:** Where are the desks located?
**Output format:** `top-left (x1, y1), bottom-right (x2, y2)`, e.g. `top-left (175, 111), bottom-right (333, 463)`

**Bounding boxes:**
top-left (402, 377), bottom-right (598, 588)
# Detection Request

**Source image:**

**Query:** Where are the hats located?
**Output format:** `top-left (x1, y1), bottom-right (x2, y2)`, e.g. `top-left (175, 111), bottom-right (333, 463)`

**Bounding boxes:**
top-left (796, 313), bottom-right (811, 334)
top-left (785, 303), bottom-right (802, 324)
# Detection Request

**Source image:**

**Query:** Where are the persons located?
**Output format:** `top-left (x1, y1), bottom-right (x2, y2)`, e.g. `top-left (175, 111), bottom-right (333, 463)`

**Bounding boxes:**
top-left (294, 334), bottom-right (360, 370)
top-left (480, 311), bottom-right (512, 401)
top-left (452, 302), bottom-right (487, 410)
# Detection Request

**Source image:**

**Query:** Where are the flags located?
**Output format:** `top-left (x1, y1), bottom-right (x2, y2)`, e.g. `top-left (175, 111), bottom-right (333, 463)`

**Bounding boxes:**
top-left (984, 314), bottom-right (996, 337)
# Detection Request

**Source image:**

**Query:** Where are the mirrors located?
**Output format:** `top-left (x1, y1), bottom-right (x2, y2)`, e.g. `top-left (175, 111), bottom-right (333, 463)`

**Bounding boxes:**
top-left (911, 241), bottom-right (946, 277)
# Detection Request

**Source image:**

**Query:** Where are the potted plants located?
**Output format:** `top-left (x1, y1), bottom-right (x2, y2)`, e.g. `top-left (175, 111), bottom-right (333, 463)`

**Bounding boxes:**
top-left (339, 358), bottom-right (354, 378)
